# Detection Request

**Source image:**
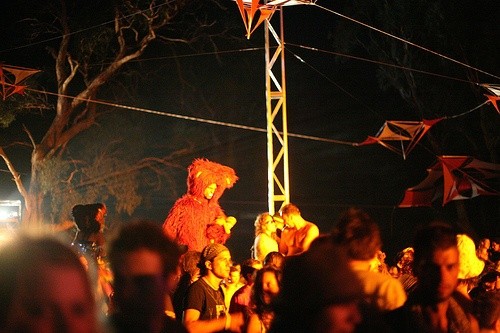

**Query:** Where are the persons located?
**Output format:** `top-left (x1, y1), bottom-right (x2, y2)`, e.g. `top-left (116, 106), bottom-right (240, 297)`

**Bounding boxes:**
top-left (0, 158), bottom-right (500, 333)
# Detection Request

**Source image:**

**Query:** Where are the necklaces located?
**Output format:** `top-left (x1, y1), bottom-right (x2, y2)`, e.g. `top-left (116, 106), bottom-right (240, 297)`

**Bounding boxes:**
top-left (198, 277), bottom-right (226, 311)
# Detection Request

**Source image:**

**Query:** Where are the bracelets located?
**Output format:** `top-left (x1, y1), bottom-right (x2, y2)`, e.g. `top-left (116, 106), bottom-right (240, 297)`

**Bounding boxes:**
top-left (225, 312), bottom-right (231, 330)
